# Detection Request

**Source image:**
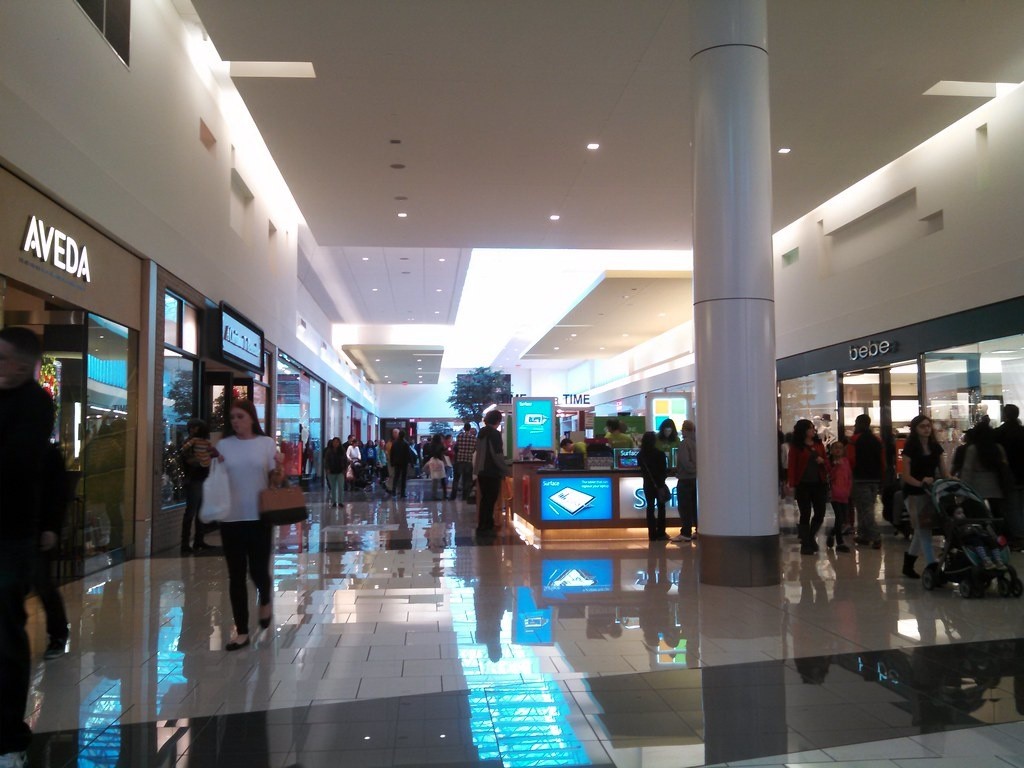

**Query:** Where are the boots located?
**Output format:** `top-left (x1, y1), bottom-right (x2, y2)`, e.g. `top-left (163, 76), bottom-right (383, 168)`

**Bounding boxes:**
top-left (902, 550), bottom-right (921, 579)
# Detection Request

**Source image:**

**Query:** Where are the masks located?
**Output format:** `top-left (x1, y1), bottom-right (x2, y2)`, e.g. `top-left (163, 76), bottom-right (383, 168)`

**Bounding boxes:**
top-left (664, 428), bottom-right (672, 437)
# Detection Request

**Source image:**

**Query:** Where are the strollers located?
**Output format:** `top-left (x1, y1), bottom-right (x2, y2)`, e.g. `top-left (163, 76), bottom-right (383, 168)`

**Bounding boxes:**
top-left (350, 458), bottom-right (374, 492)
top-left (921, 475), bottom-right (1024, 599)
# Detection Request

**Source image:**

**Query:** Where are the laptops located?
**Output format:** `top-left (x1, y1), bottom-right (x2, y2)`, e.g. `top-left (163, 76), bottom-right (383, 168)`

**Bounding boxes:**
top-left (557, 453), bottom-right (586, 471)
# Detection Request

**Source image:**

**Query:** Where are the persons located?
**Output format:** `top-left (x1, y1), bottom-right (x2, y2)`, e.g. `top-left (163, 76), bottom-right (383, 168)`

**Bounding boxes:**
top-left (88, 418), bottom-right (128, 551)
top-left (1, 328), bottom-right (72, 768)
top-left (174, 403), bottom-right (1024, 583)
top-left (211, 400), bottom-right (286, 652)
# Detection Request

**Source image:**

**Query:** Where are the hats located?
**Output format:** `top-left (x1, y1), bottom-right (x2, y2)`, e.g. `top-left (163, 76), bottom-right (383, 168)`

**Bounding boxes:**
top-left (820, 414), bottom-right (832, 421)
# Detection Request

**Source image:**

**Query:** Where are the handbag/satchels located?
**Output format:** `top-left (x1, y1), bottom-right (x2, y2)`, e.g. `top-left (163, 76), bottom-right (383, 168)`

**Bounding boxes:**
top-left (442, 454), bottom-right (453, 468)
top-left (258, 469), bottom-right (309, 525)
top-left (422, 457), bottom-right (446, 480)
top-left (776, 495), bottom-right (801, 527)
top-left (199, 458), bottom-right (232, 524)
top-left (346, 464), bottom-right (354, 480)
top-left (657, 484), bottom-right (671, 505)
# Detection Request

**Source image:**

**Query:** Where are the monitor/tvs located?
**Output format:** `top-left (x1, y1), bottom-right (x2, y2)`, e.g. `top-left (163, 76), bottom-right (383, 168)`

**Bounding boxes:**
top-left (218, 300), bottom-right (265, 376)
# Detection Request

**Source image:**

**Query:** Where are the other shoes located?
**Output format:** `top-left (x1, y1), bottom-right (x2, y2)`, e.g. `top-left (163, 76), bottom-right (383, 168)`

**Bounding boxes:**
top-left (0, 721), bottom-right (31, 768)
top-left (225, 635), bottom-right (250, 651)
top-left (872, 542), bottom-right (880, 549)
top-left (985, 559), bottom-right (1007, 570)
top-left (43, 627), bottom-right (69, 658)
top-left (853, 537), bottom-right (869, 545)
top-left (826, 531), bottom-right (834, 547)
top-left (331, 503), bottom-right (343, 508)
top-left (836, 544), bottom-right (850, 553)
top-left (648, 532), bottom-right (656, 540)
top-left (800, 536), bottom-right (819, 556)
top-left (841, 525), bottom-right (855, 534)
top-left (691, 532), bottom-right (698, 539)
top-left (258, 604), bottom-right (271, 630)
top-left (181, 537), bottom-right (216, 557)
top-left (656, 532), bottom-right (671, 540)
top-left (670, 535), bottom-right (692, 542)
